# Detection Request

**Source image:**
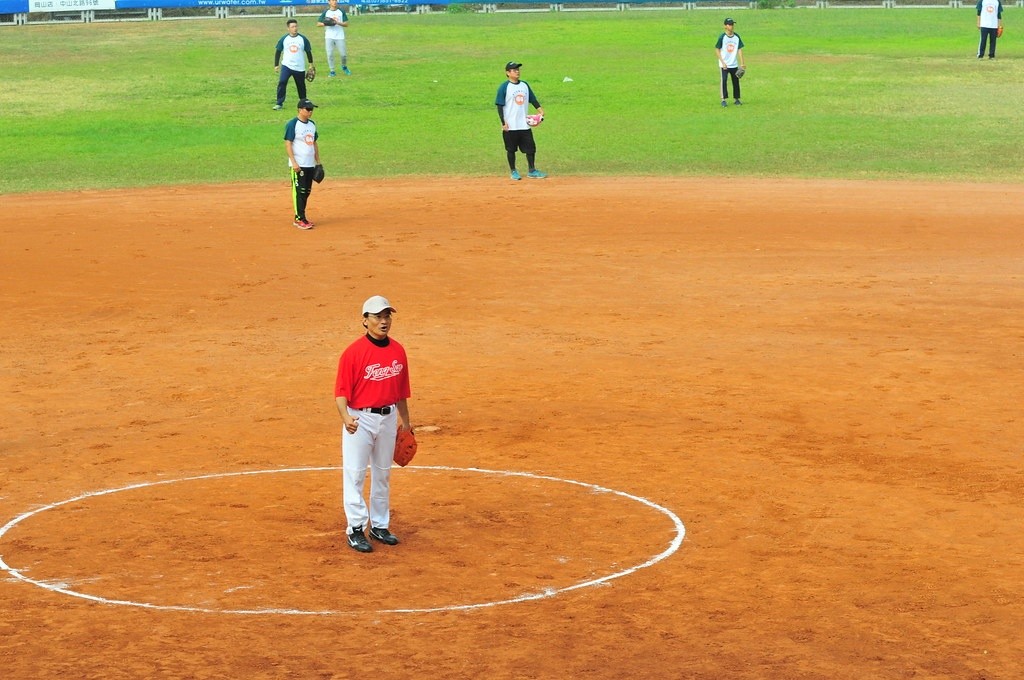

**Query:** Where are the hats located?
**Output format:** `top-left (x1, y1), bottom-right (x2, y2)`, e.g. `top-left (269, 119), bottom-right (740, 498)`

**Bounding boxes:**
top-left (506, 62), bottom-right (522, 71)
top-left (724, 18), bottom-right (736, 25)
top-left (362, 296), bottom-right (397, 315)
top-left (297, 100), bottom-right (318, 108)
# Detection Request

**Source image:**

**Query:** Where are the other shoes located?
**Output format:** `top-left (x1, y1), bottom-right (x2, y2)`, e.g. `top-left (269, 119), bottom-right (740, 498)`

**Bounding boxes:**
top-left (328, 71), bottom-right (336, 77)
top-left (342, 65), bottom-right (351, 75)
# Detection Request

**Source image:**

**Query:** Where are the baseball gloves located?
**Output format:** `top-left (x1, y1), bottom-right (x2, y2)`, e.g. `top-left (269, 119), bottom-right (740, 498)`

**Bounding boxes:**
top-left (394, 425), bottom-right (417, 467)
top-left (312, 165), bottom-right (325, 183)
top-left (306, 67), bottom-right (315, 82)
top-left (736, 65), bottom-right (747, 79)
top-left (996, 24), bottom-right (1004, 38)
top-left (323, 17), bottom-right (336, 26)
top-left (525, 114), bottom-right (544, 126)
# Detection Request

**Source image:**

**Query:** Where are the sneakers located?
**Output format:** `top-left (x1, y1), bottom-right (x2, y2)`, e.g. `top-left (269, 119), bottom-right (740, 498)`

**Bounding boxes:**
top-left (734, 100), bottom-right (742, 106)
top-left (273, 105), bottom-right (282, 110)
top-left (347, 525), bottom-right (372, 551)
top-left (368, 525), bottom-right (398, 545)
top-left (527, 170), bottom-right (547, 179)
top-left (294, 219), bottom-right (314, 230)
top-left (721, 101), bottom-right (727, 107)
top-left (511, 171), bottom-right (521, 180)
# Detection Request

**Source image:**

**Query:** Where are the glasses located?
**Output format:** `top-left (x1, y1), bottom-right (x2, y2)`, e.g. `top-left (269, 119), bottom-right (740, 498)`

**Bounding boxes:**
top-left (302, 108), bottom-right (313, 111)
top-left (366, 314), bottom-right (392, 321)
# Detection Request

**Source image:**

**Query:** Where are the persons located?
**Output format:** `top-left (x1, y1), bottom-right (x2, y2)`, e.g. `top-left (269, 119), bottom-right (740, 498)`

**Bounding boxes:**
top-left (715, 18), bottom-right (746, 108)
top-left (317, 0), bottom-right (351, 77)
top-left (495, 62), bottom-right (546, 180)
top-left (975, 0), bottom-right (1003, 61)
top-left (273, 19), bottom-right (315, 110)
top-left (335, 295), bottom-right (417, 552)
top-left (284, 99), bottom-right (324, 230)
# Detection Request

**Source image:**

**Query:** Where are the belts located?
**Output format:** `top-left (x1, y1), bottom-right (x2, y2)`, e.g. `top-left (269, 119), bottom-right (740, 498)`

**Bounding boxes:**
top-left (360, 404), bottom-right (397, 416)
